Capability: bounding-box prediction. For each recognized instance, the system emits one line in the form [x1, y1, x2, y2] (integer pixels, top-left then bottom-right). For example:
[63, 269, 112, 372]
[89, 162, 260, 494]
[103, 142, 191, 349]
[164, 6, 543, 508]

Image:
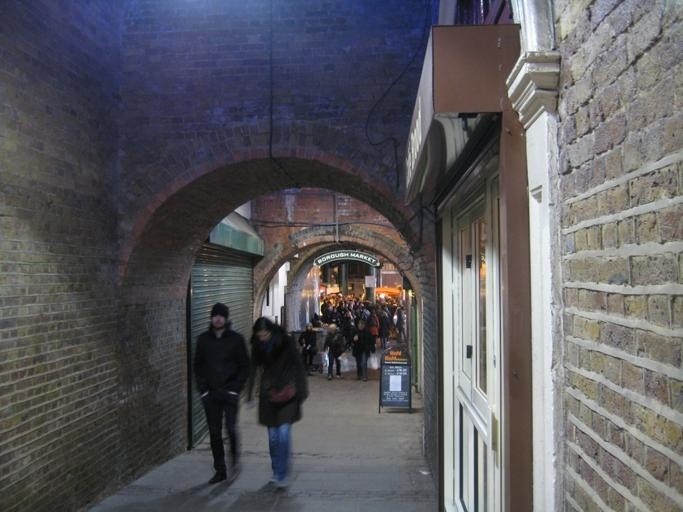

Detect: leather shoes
[210, 471, 227, 483]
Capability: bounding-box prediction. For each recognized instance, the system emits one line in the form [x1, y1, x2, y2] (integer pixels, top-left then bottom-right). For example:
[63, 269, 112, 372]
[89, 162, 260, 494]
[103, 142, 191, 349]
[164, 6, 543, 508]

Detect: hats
[211, 302, 229, 319]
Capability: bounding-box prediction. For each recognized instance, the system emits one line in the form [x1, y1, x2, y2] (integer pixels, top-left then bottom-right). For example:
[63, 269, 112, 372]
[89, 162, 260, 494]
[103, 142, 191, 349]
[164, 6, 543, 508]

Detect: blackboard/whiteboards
[380, 361, 410, 406]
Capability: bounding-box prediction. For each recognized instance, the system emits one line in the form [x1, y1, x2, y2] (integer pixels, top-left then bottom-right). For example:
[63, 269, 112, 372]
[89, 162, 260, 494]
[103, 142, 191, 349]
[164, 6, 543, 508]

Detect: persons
[192, 302, 250, 486]
[296, 321, 318, 367]
[244, 316, 309, 490]
[311, 291, 406, 382]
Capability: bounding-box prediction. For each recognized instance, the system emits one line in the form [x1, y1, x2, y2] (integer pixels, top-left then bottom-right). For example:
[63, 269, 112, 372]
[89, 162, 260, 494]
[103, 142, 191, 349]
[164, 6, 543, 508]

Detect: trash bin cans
[292, 328, 324, 371]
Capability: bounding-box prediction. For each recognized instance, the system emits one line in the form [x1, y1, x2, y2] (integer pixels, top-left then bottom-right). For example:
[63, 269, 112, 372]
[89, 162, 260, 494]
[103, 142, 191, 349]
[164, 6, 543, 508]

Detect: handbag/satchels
[268, 384, 297, 403]
